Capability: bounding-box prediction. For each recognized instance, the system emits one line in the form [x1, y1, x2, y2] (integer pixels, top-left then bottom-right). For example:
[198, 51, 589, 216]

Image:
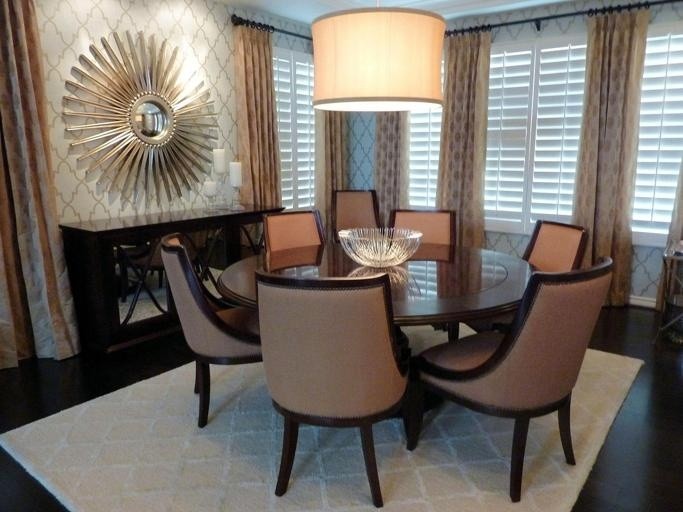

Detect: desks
[62, 201, 282, 368]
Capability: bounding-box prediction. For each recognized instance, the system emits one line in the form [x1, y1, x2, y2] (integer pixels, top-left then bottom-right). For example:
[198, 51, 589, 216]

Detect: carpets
[0, 343, 645, 511]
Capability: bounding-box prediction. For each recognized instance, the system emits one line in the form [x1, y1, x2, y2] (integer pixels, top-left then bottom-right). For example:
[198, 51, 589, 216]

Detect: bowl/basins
[338, 228, 424, 269]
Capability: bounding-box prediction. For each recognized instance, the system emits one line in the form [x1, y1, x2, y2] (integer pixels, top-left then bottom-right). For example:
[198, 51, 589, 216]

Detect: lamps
[308, 0, 451, 118]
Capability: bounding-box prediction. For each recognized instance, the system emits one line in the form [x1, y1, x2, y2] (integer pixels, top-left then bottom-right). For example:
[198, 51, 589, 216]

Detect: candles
[202, 148, 242, 197]
